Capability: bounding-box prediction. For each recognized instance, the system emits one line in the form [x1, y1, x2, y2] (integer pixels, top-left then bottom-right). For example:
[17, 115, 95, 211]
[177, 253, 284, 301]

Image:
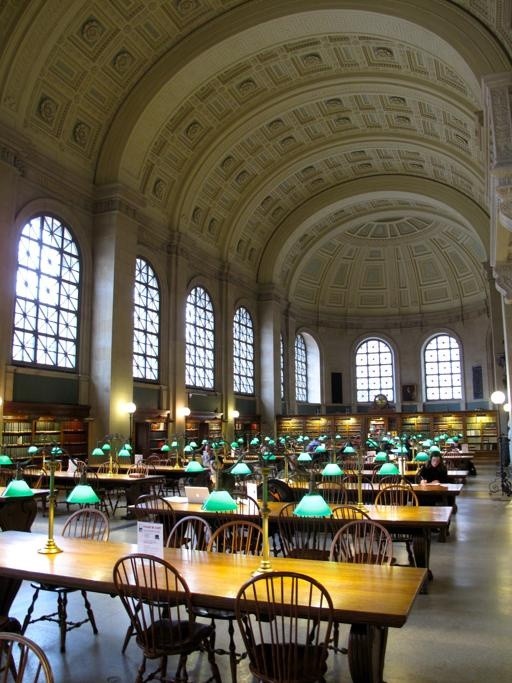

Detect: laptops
[183, 486, 210, 503]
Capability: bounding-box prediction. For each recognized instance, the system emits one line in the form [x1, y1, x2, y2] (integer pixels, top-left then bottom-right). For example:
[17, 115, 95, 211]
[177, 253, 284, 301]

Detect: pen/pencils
[421, 476, 424, 480]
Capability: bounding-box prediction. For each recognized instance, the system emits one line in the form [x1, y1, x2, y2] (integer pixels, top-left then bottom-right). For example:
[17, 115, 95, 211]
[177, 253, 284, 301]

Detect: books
[404, 414, 498, 449]
[3, 421, 60, 485]
[279, 416, 385, 445]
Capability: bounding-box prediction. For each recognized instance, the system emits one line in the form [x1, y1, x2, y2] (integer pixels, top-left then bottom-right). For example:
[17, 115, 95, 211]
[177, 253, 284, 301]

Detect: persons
[190, 454, 235, 552]
[376, 442, 391, 453]
[418, 449, 448, 485]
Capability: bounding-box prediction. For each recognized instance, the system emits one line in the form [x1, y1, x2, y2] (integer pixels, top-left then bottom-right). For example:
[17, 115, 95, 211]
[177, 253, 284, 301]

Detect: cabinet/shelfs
[1, 401, 92, 485]
[136, 407, 260, 458]
[278, 409, 500, 458]
